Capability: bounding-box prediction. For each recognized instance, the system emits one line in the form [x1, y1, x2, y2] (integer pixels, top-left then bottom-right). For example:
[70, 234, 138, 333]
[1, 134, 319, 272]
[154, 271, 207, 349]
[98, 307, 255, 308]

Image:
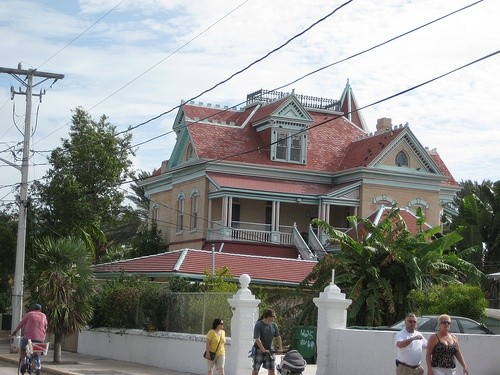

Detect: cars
[390, 314, 497, 335]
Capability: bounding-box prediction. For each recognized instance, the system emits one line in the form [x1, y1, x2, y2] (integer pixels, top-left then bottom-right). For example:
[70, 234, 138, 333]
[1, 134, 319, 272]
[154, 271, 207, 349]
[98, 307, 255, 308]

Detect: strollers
[263, 349, 306, 375]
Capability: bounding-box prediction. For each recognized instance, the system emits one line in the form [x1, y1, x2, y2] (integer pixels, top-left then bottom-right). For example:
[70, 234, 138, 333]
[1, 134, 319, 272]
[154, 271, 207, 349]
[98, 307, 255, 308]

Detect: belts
[396, 359, 420, 369]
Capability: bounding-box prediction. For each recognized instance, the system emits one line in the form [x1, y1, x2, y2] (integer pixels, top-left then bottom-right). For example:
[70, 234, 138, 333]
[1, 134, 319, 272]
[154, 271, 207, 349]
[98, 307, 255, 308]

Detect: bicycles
[10, 334, 42, 375]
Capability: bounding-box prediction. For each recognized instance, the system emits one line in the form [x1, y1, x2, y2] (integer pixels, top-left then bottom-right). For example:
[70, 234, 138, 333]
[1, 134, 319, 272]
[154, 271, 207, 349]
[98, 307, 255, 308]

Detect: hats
[33, 304, 41, 310]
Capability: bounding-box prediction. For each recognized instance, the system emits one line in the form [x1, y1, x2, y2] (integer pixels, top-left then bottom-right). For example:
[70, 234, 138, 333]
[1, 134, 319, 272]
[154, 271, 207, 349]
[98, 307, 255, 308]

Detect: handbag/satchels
[204, 351, 216, 361]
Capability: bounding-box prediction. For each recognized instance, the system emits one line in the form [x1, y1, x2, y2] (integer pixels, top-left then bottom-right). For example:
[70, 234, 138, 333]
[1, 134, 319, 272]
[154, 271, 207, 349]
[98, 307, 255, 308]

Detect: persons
[252, 309, 282, 375]
[206, 318, 226, 375]
[10, 304, 48, 373]
[396, 314, 428, 375]
[426, 315, 469, 375]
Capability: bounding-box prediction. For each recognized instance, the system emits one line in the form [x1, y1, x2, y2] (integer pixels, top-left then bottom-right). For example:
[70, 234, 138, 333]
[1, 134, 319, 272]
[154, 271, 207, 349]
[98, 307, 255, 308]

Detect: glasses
[220, 323, 224, 325]
[409, 320, 416, 323]
[440, 321, 451, 325]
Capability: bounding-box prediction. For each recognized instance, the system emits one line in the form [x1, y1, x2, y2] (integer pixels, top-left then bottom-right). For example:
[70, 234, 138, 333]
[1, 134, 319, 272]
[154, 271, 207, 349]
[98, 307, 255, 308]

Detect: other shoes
[20, 364, 27, 373]
